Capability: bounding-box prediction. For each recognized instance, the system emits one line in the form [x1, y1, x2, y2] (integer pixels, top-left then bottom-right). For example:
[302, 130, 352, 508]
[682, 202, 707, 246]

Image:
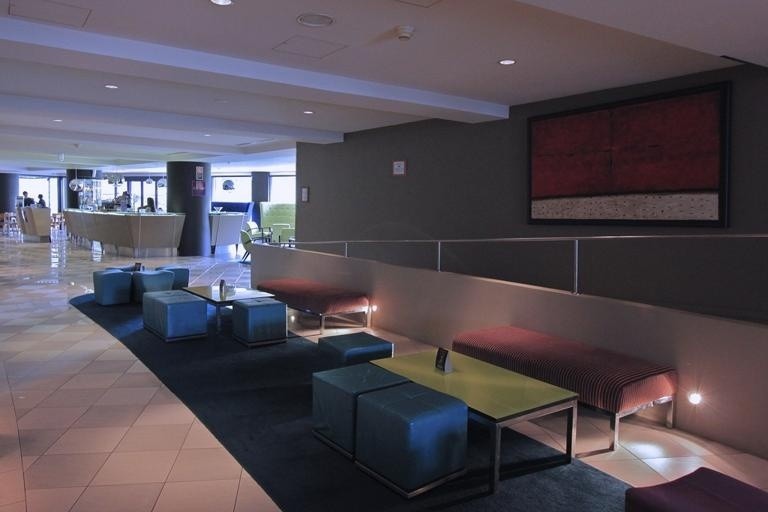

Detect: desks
[15, 207, 28, 235]
[22, 207, 50, 243]
[63, 208, 185, 258]
[209, 212, 243, 253]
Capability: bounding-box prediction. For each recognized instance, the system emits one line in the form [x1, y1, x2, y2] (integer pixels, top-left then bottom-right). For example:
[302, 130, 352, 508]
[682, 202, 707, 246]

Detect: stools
[142, 290, 192, 333]
[155, 265, 189, 291]
[310, 362, 410, 461]
[354, 381, 468, 498]
[231, 297, 287, 348]
[132, 270, 174, 305]
[105, 266, 145, 272]
[318, 332, 394, 368]
[152, 295, 208, 343]
[93, 269, 133, 306]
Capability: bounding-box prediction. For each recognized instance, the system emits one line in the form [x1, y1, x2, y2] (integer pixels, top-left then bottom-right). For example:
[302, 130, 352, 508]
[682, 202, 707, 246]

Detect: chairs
[278, 228, 295, 245]
[246, 221, 271, 243]
[271, 224, 290, 243]
[240, 230, 272, 263]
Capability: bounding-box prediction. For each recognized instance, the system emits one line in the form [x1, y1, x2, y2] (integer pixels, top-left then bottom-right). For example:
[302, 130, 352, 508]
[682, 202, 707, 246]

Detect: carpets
[69, 288, 631, 512]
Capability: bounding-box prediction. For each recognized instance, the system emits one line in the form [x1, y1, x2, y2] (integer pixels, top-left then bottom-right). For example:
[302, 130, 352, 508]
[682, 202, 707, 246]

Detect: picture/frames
[526, 85, 731, 229]
[390, 159, 407, 177]
[300, 185, 310, 203]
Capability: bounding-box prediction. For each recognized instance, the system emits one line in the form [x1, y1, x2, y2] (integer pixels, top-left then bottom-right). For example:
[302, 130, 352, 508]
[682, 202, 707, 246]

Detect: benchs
[451, 325, 676, 450]
[257, 278, 371, 334]
[625, 467, 767, 512]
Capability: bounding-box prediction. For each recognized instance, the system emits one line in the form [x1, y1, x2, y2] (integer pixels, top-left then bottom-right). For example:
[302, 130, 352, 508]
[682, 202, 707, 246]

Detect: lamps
[158, 172, 168, 187]
[223, 180, 236, 191]
[69, 168, 83, 192]
[146, 174, 152, 184]
[116, 175, 125, 185]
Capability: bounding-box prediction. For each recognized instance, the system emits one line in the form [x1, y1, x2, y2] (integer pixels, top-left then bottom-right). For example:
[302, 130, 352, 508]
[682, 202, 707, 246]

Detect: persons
[23, 191, 33, 205]
[145, 198, 158, 213]
[38, 194, 46, 207]
